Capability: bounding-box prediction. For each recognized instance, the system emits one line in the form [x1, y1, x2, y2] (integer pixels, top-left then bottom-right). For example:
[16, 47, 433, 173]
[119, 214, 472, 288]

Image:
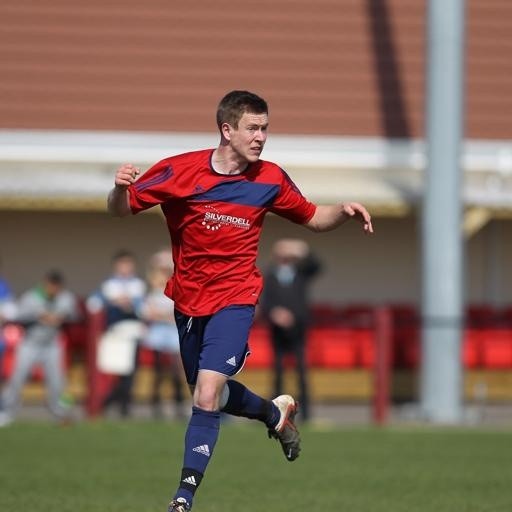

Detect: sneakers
[167, 501, 191, 512]
[268, 394, 302, 460]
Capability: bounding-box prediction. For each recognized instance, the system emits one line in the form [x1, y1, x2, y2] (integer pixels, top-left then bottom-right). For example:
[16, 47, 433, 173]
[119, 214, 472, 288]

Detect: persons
[98, 251, 186, 431]
[261, 239, 323, 426]
[1, 270, 79, 427]
[106, 89, 376, 512]
